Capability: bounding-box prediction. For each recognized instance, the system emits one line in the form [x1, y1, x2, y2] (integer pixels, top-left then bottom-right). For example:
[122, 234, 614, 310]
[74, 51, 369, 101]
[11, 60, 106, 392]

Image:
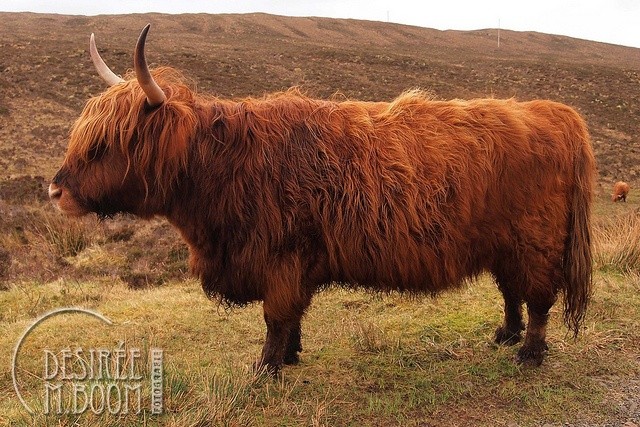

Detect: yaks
[47, 25, 594, 373]
[612, 180, 628, 202]
